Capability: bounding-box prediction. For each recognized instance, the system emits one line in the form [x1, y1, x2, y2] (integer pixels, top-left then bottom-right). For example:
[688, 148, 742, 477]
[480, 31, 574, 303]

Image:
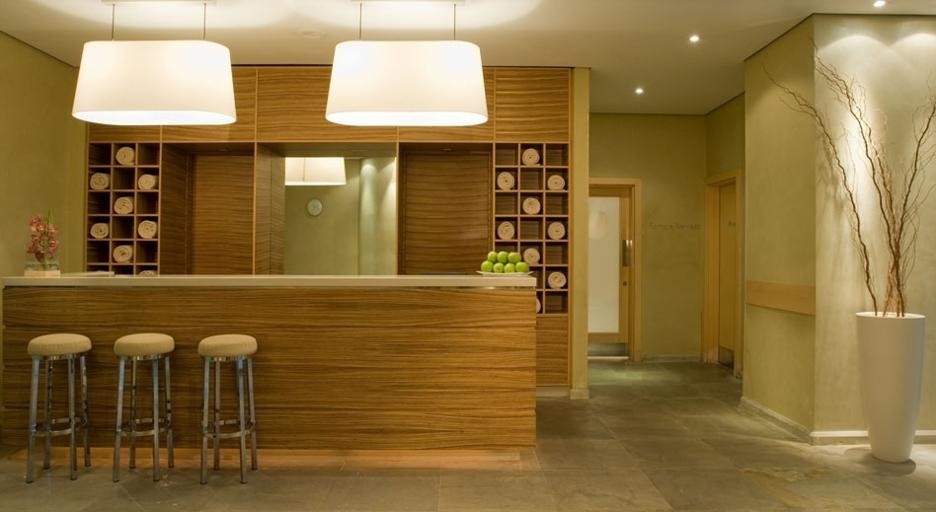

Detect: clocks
[306, 197, 323, 216]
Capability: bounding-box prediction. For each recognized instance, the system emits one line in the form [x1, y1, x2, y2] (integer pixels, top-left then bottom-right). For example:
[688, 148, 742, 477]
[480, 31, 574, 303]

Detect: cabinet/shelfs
[490, 142, 573, 399]
[83, 143, 194, 274]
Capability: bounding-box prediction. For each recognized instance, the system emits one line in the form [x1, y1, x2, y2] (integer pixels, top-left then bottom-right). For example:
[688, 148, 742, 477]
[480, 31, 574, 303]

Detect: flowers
[22, 208, 60, 270]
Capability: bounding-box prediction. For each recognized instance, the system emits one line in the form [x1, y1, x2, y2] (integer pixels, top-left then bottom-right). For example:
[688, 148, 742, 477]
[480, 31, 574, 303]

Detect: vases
[857, 310, 926, 464]
[23, 263, 59, 278]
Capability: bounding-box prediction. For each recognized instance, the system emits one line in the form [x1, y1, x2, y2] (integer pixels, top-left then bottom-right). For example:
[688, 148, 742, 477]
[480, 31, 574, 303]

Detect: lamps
[282, 157, 347, 186]
[69, 2, 237, 125]
[323, 2, 488, 127]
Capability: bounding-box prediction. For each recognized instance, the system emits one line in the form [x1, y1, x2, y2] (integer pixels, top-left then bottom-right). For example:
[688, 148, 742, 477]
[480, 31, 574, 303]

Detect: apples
[480, 250, 529, 273]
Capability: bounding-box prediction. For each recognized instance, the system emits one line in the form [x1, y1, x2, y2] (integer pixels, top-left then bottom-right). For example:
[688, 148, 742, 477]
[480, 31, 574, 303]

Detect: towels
[90, 147, 161, 273]
[496, 147, 568, 312]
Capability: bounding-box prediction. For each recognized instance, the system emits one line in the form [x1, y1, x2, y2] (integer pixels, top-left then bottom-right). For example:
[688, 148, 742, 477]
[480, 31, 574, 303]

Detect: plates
[474, 269, 536, 277]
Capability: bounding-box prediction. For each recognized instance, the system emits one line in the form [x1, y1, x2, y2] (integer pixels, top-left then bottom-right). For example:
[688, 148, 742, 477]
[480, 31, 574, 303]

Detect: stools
[24, 333, 257, 484]
